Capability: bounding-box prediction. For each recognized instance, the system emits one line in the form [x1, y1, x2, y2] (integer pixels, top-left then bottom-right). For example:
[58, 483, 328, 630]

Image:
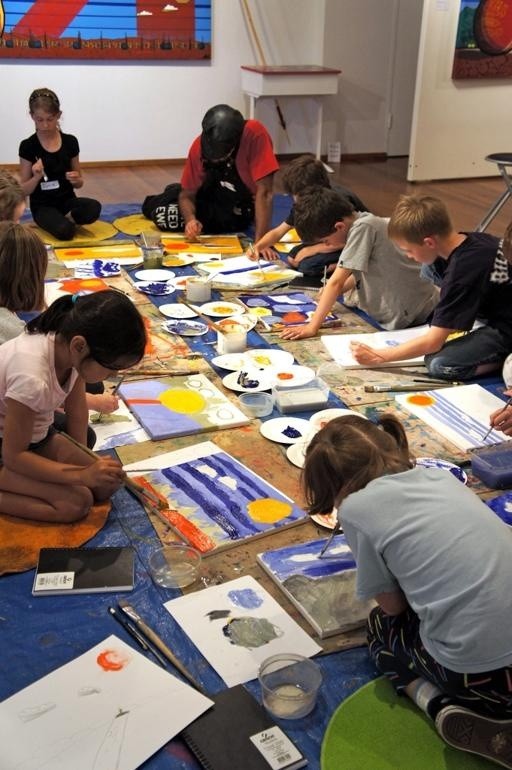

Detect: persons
[0, 219, 104, 452]
[298, 410, 512, 770]
[490, 222, 512, 438]
[142, 105, 282, 261]
[1, 170, 26, 225]
[17, 89, 101, 241]
[0, 289, 146, 524]
[349, 190, 512, 380]
[246, 156, 371, 277]
[278, 186, 441, 342]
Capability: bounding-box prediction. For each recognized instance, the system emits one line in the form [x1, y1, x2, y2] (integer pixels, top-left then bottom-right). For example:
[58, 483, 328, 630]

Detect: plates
[134, 279, 176, 296]
[160, 318, 210, 337]
[135, 268, 175, 281]
[308, 407, 369, 429]
[408, 456, 469, 486]
[200, 300, 245, 318]
[167, 276, 210, 291]
[211, 313, 260, 336]
[285, 442, 313, 469]
[211, 351, 261, 373]
[243, 350, 295, 370]
[261, 364, 317, 390]
[308, 503, 345, 533]
[159, 254, 194, 266]
[259, 415, 315, 444]
[221, 369, 276, 394]
[158, 302, 203, 319]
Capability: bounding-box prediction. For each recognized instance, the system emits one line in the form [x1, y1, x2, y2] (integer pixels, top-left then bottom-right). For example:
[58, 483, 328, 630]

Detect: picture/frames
[0, 0, 212, 62]
[451, 0, 512, 80]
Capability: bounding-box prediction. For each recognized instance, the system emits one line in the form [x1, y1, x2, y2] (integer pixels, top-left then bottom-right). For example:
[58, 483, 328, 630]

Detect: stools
[476, 149, 512, 242]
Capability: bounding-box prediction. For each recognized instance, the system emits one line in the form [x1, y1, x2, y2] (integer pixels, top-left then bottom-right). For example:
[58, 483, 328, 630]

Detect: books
[31, 547, 135, 596]
[184, 685, 309, 768]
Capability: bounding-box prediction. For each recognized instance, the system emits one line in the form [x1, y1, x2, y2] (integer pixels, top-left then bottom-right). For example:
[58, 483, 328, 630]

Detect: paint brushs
[249, 242, 267, 277]
[482, 398, 512, 441]
[211, 285, 267, 293]
[97, 376, 125, 423]
[108, 285, 129, 295]
[204, 272, 220, 284]
[180, 300, 228, 335]
[413, 379, 460, 385]
[195, 235, 206, 244]
[107, 606, 148, 650]
[361, 345, 387, 360]
[118, 599, 205, 694]
[317, 521, 341, 558]
[118, 370, 199, 376]
[34, 155, 49, 182]
[323, 265, 327, 288]
[126, 263, 144, 272]
[260, 330, 283, 334]
[141, 232, 147, 247]
[57, 429, 169, 511]
[365, 385, 453, 393]
[43, 279, 58, 282]
[126, 622, 168, 667]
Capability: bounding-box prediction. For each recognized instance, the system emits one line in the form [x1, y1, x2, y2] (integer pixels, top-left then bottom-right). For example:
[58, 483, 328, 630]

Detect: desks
[238, 65, 342, 173]
